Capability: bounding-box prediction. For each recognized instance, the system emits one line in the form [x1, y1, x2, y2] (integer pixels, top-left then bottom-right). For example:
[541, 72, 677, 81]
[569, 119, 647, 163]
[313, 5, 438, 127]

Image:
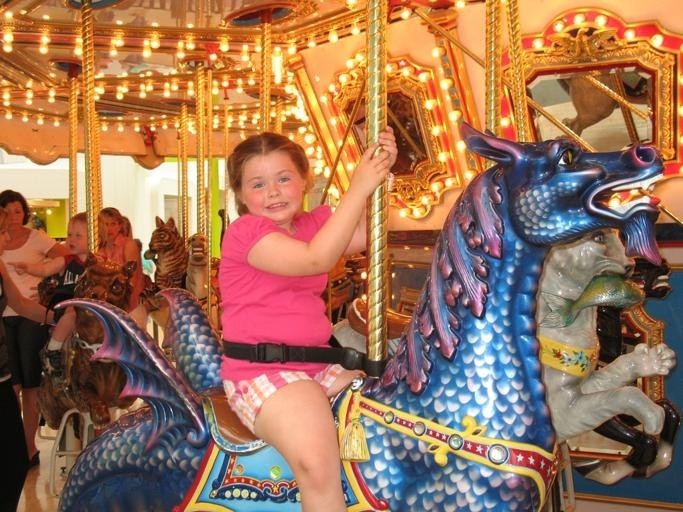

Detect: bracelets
[386, 170, 396, 190]
[44, 308, 50, 324]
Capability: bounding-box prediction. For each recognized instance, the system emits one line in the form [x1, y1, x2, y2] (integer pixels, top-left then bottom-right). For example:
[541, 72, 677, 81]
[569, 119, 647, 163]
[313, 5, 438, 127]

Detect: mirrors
[490, 7, 683, 205]
[326, 48, 462, 219]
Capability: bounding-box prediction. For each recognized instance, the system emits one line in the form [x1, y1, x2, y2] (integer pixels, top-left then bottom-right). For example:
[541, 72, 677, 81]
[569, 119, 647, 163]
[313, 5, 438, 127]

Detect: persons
[7, 212, 108, 373]
[0, 189, 69, 464]
[121, 216, 142, 252]
[216, 125, 400, 512]
[1, 211, 62, 512]
[98, 208, 147, 328]
